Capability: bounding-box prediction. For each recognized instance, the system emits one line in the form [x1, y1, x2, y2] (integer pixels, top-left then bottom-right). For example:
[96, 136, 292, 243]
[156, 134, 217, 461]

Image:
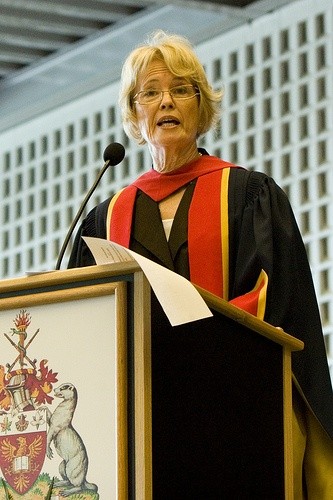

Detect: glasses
[131, 85, 200, 105]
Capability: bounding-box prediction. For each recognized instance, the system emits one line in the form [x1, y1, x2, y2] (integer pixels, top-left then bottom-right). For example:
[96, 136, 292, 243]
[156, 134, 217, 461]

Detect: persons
[66, 28, 333, 500]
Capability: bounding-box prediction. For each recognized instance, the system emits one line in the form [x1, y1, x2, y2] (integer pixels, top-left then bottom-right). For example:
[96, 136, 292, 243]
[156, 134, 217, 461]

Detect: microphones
[56, 142, 126, 271]
